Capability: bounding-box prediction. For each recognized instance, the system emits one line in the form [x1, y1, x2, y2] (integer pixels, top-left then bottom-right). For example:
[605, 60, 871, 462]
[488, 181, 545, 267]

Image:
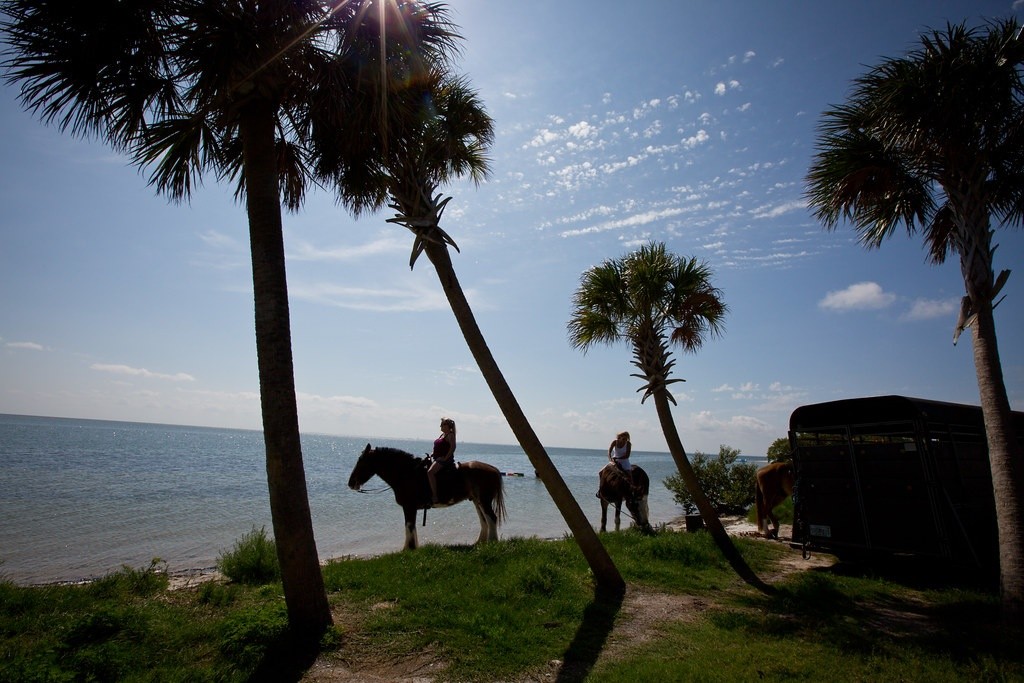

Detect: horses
[755, 459, 793, 538]
[598, 462, 650, 534]
[347, 442, 512, 552]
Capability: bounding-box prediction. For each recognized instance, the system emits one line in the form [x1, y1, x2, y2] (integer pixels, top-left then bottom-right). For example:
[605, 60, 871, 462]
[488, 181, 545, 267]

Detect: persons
[607, 431, 633, 485]
[427, 417, 457, 504]
